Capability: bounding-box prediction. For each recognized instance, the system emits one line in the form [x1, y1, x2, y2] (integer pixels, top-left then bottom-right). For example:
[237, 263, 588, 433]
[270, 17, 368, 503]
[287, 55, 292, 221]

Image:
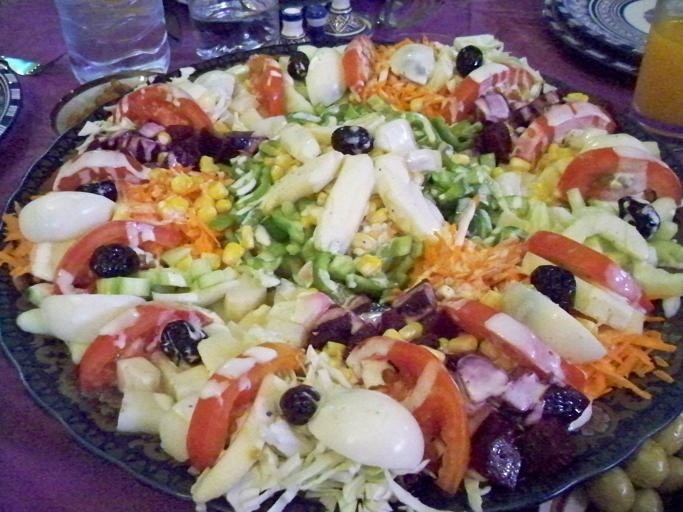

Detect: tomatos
[16, 35, 683, 492]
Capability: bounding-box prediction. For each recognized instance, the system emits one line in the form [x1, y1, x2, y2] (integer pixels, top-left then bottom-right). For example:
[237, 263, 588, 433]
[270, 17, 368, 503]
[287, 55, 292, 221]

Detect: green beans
[586, 414, 682, 509]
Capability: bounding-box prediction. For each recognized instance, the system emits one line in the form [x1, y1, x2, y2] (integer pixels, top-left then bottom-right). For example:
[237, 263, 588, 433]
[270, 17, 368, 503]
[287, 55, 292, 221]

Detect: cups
[626, 0, 683, 138]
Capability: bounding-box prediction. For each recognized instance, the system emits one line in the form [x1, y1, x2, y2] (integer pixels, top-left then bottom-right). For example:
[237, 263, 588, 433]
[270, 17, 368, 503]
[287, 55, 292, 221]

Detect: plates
[543, 0, 654, 73]
[0, 38, 683, 512]
